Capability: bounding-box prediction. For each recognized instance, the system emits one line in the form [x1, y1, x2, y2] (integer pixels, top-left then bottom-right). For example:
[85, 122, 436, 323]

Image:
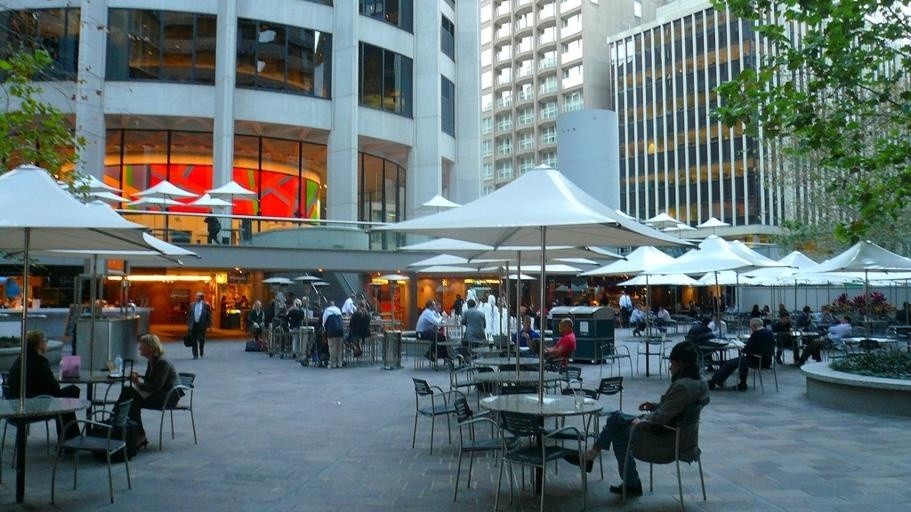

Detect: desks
[271, 317, 402, 367]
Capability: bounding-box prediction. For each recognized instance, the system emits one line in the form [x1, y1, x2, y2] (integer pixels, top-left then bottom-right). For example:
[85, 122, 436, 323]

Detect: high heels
[136, 437, 149, 452]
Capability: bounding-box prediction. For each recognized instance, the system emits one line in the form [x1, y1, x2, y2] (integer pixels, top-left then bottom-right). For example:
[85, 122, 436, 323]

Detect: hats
[661, 340, 699, 366]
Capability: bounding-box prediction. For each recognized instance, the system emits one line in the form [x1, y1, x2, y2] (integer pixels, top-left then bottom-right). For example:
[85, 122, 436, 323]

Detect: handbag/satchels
[184, 329, 193, 347]
[246, 338, 260, 352]
[89, 414, 140, 465]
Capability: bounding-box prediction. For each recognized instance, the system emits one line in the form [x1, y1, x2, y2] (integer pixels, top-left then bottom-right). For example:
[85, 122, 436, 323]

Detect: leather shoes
[707, 379, 725, 389]
[559, 446, 595, 476]
[732, 383, 748, 391]
[608, 481, 646, 498]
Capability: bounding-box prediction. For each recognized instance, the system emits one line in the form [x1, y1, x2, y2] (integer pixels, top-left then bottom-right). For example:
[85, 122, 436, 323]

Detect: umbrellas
[53, 174, 257, 246]
[0, 162, 166, 413]
[18, 200, 200, 375]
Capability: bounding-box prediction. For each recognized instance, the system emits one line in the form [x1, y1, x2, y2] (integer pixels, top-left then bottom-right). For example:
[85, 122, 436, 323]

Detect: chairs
[412, 310, 784, 393]
[0, 372, 197, 505]
[413, 378, 472, 456]
[768, 311, 910, 378]
[618, 399, 707, 510]
[454, 376, 624, 511]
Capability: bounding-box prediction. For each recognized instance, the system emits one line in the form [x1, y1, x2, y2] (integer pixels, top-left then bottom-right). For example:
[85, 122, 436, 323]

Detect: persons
[221, 292, 372, 370]
[107, 333, 185, 450]
[7, 329, 81, 452]
[187, 293, 212, 361]
[560, 341, 709, 496]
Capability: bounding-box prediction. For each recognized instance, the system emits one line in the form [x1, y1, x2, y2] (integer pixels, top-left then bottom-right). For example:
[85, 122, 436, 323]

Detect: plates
[520, 346, 530, 352]
[109, 374, 121, 377]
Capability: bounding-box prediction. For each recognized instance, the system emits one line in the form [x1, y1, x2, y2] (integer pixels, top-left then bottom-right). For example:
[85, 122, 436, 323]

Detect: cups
[443, 308, 463, 324]
[32, 299, 41, 310]
[575, 391, 586, 407]
[489, 343, 494, 348]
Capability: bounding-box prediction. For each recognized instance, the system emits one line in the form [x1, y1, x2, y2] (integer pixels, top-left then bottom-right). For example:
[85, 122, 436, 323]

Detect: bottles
[116, 353, 122, 372]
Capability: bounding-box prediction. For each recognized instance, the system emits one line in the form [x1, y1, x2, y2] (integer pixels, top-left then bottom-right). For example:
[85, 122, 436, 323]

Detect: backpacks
[212, 217, 221, 234]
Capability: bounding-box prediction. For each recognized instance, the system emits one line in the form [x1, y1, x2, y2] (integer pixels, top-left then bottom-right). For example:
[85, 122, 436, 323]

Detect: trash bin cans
[383, 330, 402, 369]
[547, 306, 615, 364]
[724, 306, 738, 315]
[300, 326, 315, 355]
